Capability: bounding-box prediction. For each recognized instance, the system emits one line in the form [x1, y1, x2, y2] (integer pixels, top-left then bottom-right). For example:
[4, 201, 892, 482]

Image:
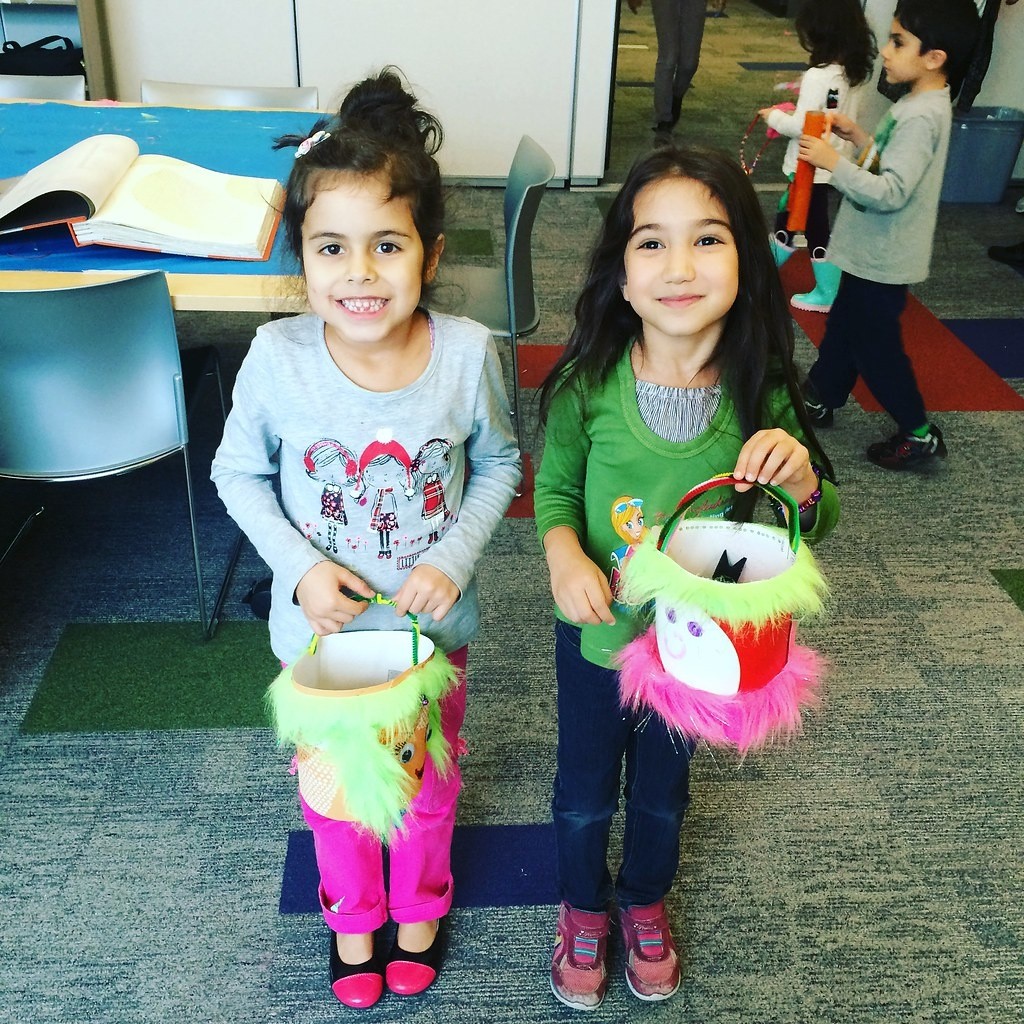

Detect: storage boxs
[938, 105, 1023, 203]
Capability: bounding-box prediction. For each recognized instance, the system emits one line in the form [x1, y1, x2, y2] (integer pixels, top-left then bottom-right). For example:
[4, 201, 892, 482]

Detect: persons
[210, 65, 523, 1006]
[785, 1, 980, 468]
[626, 0, 725, 143]
[532, 148, 838, 1010]
[757, 1, 877, 314]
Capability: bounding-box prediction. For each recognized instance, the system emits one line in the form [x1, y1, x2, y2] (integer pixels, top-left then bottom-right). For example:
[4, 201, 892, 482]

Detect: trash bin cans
[940, 104, 1024, 206]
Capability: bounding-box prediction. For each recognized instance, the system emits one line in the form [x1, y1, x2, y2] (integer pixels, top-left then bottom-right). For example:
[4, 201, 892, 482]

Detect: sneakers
[548, 901, 613, 1009]
[798, 385, 836, 430]
[617, 898, 681, 1001]
[867, 421, 948, 469]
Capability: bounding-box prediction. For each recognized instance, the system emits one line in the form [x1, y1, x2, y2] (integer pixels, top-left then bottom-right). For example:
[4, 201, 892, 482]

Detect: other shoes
[329, 926, 386, 1009]
[650, 94, 685, 146]
[385, 916, 448, 993]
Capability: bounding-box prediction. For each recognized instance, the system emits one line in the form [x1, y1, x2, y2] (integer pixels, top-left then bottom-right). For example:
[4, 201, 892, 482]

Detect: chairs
[0, 271, 251, 641]
[0, 74, 86, 103]
[418, 134, 557, 500]
[139, 78, 320, 112]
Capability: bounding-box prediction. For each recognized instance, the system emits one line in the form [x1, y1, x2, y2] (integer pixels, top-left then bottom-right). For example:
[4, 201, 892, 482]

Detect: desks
[0, 97, 344, 313]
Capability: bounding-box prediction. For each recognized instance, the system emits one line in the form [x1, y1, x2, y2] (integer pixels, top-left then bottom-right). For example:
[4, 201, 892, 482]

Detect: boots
[766, 232, 797, 269]
[789, 255, 844, 313]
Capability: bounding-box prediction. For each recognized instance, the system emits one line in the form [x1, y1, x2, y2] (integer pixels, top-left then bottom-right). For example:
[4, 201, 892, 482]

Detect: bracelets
[769, 462, 823, 517]
